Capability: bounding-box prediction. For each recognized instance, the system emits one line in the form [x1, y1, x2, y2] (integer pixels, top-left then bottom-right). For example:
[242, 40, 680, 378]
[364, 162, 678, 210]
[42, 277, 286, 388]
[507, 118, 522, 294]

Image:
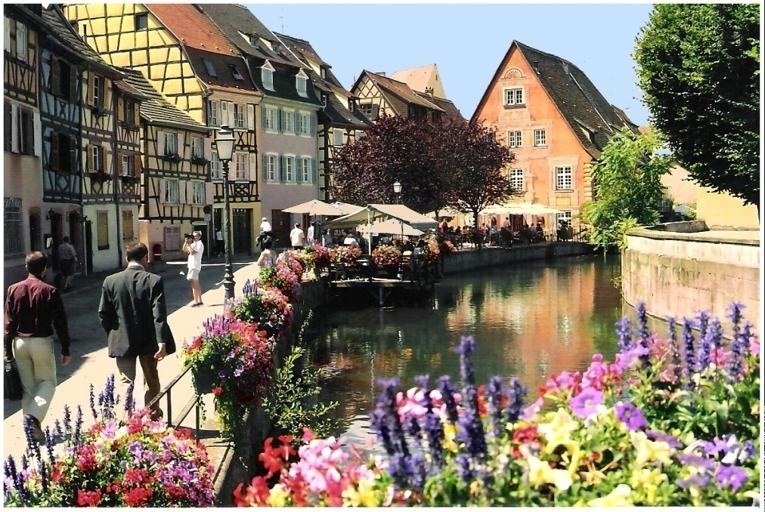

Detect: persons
[97, 241, 177, 423]
[4, 251, 74, 447]
[182, 229, 207, 308]
[214, 229, 227, 259]
[255, 216, 362, 252]
[55, 235, 80, 289]
[438, 217, 569, 247]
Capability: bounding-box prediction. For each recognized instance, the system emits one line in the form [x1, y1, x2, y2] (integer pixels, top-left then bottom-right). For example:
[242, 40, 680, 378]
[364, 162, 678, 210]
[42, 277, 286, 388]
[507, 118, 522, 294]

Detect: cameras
[184, 234, 194, 239]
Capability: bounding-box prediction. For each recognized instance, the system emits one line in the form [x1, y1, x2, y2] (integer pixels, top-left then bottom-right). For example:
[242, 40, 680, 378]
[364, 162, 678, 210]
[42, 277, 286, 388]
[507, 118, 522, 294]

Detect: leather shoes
[23, 414, 45, 446]
[148, 409, 163, 421]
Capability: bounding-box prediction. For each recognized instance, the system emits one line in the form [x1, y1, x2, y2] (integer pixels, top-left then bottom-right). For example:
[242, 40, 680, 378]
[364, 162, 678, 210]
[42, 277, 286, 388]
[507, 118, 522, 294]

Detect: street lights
[213, 124, 239, 310]
[393, 177, 402, 253]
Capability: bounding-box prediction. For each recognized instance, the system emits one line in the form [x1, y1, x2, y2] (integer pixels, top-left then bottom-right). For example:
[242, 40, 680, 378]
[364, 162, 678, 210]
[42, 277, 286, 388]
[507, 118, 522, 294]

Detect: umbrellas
[280, 197, 346, 244]
[309, 200, 366, 217]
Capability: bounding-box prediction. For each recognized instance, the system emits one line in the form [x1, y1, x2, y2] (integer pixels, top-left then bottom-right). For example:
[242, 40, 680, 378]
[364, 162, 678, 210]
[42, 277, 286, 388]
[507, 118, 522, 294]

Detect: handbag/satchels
[4, 355, 22, 400]
